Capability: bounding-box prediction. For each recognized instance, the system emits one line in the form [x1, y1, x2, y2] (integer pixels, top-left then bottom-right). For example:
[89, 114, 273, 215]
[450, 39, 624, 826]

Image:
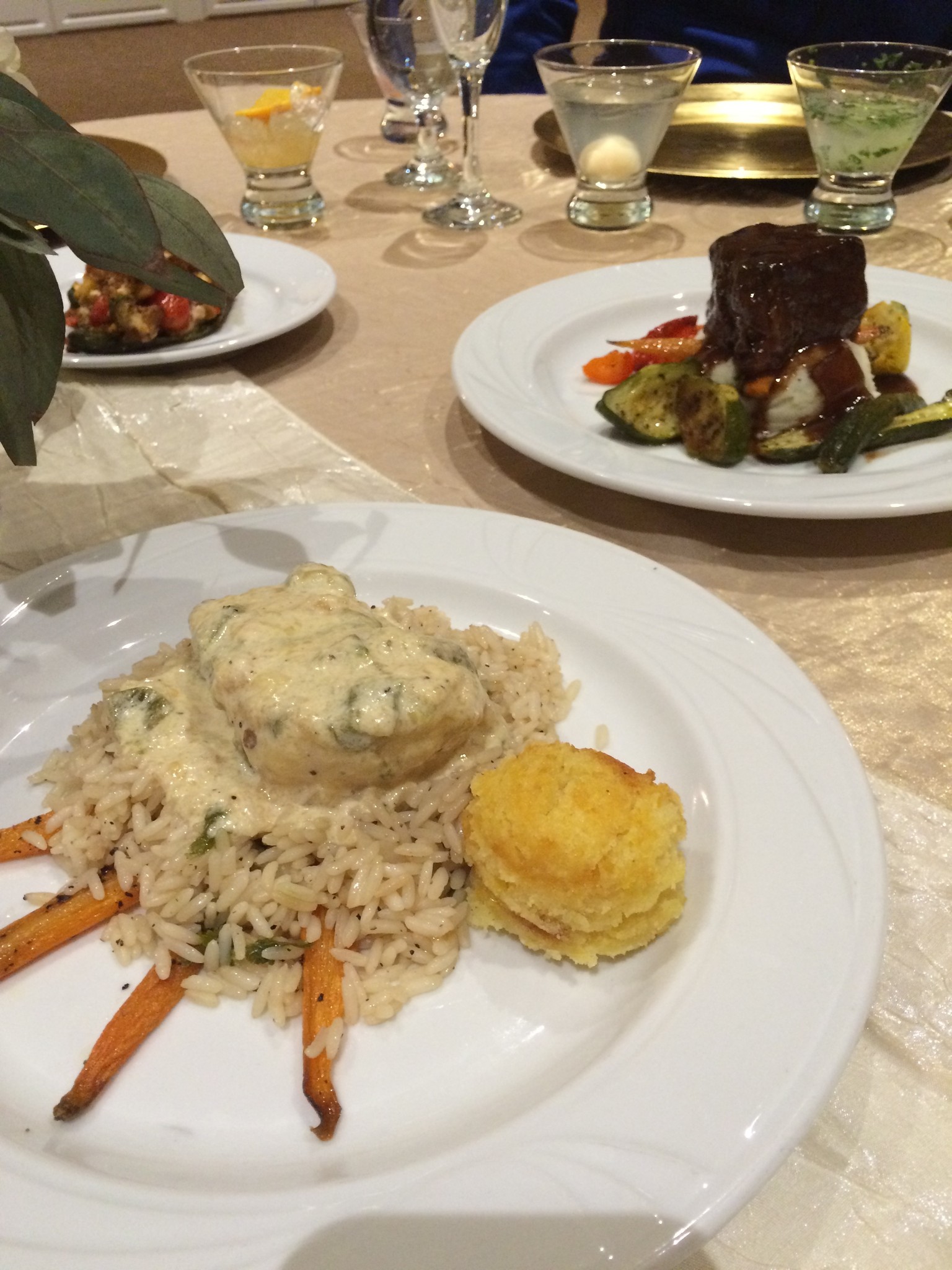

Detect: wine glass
[365, 0, 480, 191]
[417, 0, 525, 231]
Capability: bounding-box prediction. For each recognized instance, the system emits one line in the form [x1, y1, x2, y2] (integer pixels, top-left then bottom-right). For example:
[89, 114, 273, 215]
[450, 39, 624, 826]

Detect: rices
[22, 563, 576, 1025]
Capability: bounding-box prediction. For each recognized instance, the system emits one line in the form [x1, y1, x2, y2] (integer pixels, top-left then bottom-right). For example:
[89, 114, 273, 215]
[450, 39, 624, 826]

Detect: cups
[787, 41, 951, 235]
[345, 2, 446, 138]
[533, 39, 701, 232]
[183, 44, 345, 231]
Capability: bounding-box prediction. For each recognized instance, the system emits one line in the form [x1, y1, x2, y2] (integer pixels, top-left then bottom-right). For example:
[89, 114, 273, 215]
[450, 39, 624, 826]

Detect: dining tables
[4, 92, 950, 1269]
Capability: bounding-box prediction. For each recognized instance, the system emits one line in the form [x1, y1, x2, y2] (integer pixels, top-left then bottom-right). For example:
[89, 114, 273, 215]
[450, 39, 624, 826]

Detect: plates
[0, 497, 886, 1267]
[535, 82, 952, 182]
[45, 233, 338, 370]
[451, 254, 952, 518]
